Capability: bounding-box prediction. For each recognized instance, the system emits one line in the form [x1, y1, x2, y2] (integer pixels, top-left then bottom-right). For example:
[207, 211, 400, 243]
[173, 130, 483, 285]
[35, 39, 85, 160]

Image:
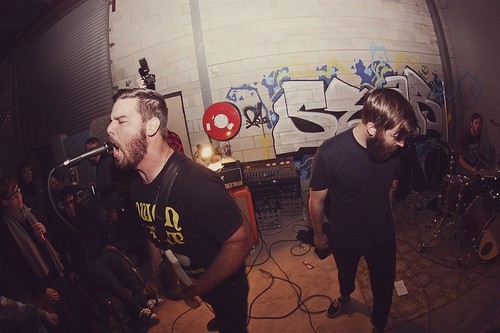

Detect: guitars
[163, 250, 199, 309]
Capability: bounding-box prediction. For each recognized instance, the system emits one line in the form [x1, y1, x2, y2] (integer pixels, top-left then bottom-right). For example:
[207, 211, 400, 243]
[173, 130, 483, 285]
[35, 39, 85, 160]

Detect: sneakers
[371, 326, 385, 333]
[327, 294, 351, 317]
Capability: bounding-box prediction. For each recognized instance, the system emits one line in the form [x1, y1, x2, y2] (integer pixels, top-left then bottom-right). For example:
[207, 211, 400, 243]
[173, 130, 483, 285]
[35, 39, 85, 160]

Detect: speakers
[220, 184, 258, 245]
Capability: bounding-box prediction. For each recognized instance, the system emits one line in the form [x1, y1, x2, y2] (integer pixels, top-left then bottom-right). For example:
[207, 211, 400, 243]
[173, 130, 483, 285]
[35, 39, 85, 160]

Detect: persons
[454, 113, 487, 192]
[0, 131, 185, 333]
[106, 88, 252, 333]
[309, 87, 417, 333]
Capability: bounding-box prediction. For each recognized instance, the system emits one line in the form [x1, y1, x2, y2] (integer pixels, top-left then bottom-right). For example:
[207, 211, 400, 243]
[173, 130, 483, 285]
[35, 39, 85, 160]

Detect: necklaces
[165, 147, 170, 163]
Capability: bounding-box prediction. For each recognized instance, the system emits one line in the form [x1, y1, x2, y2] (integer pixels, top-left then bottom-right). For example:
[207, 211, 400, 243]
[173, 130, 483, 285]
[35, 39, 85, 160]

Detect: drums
[461, 190, 500, 265]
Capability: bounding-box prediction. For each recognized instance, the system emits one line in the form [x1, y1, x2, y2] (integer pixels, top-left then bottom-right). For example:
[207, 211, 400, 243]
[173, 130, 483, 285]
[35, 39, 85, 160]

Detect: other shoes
[137, 307, 157, 322]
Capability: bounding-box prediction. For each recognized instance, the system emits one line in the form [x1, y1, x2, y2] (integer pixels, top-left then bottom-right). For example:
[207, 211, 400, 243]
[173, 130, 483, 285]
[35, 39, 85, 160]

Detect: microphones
[55, 141, 113, 169]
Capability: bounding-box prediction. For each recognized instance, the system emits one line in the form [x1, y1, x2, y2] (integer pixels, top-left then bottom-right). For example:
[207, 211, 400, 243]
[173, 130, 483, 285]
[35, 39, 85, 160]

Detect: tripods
[419, 170, 472, 268]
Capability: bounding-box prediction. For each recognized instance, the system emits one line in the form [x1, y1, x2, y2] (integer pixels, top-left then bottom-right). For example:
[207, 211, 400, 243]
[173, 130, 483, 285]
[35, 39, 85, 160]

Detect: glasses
[0, 187, 21, 201]
